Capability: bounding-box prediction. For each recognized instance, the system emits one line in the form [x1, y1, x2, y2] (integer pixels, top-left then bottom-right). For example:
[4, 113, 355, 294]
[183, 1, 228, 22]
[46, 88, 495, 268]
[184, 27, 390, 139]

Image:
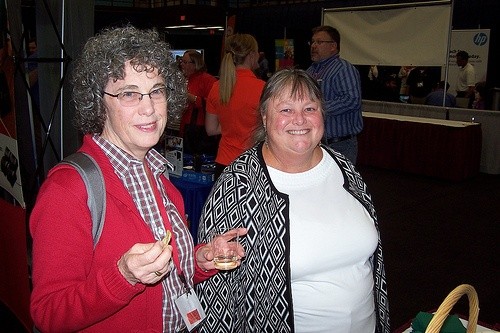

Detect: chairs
[456, 97, 470, 107]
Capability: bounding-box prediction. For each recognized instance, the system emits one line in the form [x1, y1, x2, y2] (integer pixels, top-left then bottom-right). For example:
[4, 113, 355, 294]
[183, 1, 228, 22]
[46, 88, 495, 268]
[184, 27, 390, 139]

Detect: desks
[356, 110, 484, 186]
[390, 307, 500, 333]
[169, 172, 215, 245]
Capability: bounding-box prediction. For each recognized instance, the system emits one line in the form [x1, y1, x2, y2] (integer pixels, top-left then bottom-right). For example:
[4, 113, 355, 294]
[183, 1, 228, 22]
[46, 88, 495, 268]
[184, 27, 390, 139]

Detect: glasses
[182, 60, 194, 65]
[102, 86, 176, 107]
[308, 40, 337, 46]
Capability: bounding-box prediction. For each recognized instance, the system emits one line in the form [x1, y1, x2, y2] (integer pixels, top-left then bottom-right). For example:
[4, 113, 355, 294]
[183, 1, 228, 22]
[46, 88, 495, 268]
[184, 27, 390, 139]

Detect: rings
[155, 271, 163, 276]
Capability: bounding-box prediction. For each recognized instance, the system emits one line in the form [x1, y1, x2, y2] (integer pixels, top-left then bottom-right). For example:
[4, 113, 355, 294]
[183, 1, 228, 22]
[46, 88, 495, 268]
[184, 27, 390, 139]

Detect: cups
[210, 228, 239, 270]
[201, 156, 215, 174]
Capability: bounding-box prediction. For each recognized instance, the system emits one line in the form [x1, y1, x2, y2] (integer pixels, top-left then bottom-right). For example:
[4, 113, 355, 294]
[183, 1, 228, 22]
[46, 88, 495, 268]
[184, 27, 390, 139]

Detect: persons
[30, 27, 247, 333]
[205, 34, 269, 180]
[192, 66, 391, 333]
[26, 40, 42, 115]
[304, 26, 364, 169]
[173, 50, 220, 155]
[398, 48, 478, 107]
